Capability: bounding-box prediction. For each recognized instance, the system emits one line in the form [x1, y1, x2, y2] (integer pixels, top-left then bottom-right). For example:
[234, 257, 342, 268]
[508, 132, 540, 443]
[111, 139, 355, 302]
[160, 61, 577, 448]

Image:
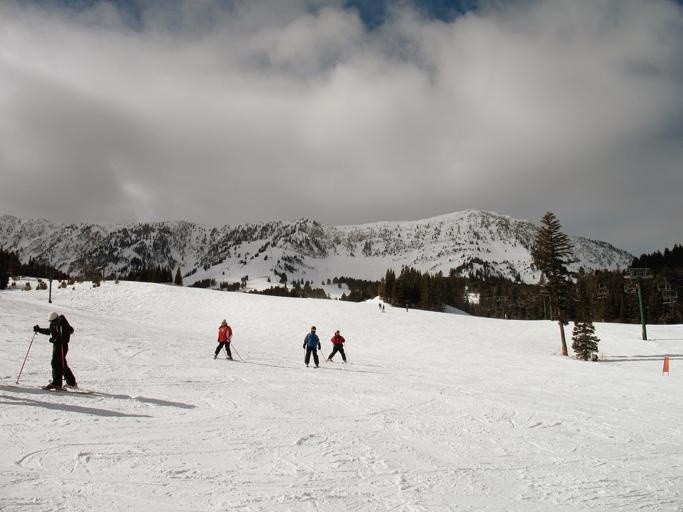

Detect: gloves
[48, 337, 57, 343]
[33, 326, 41, 333]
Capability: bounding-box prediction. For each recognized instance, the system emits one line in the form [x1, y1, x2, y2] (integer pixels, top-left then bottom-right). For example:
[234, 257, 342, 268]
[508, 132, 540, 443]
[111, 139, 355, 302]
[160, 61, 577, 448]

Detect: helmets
[48, 311, 58, 323]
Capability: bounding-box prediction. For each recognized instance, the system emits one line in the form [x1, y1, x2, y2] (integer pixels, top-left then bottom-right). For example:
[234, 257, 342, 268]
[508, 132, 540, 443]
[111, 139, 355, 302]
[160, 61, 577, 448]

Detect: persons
[33, 312, 76, 389]
[328, 330, 346, 362]
[405, 303, 409, 312]
[303, 326, 321, 367]
[378, 303, 385, 312]
[213, 319, 232, 360]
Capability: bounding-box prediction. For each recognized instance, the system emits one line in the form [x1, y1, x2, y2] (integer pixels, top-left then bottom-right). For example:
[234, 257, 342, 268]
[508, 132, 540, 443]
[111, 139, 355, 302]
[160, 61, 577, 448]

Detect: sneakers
[45, 382, 77, 390]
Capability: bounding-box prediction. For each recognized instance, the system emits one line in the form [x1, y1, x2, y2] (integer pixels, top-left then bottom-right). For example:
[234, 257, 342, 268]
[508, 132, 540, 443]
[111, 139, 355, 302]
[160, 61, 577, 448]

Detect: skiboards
[40, 380, 96, 394]
[305, 365, 321, 369]
